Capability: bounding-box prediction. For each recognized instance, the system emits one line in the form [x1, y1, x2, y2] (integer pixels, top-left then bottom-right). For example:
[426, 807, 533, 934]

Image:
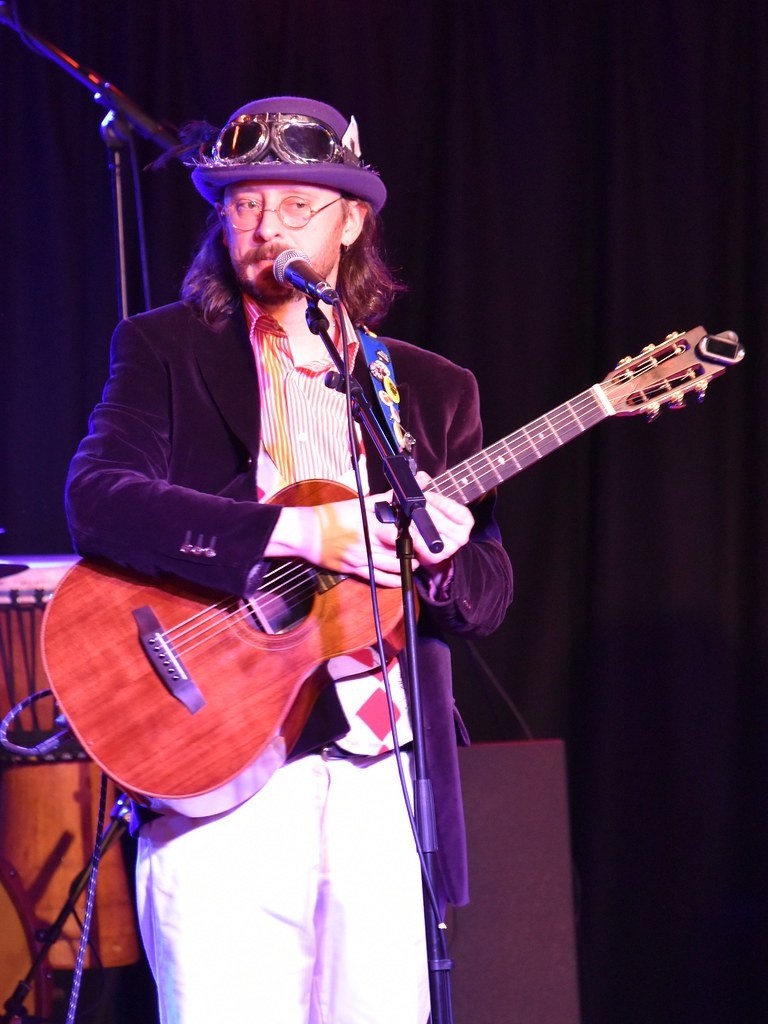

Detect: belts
[309, 740, 414, 761]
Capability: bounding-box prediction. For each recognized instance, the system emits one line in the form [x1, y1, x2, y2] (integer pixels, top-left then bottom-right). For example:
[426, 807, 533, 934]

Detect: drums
[0, 551, 142, 972]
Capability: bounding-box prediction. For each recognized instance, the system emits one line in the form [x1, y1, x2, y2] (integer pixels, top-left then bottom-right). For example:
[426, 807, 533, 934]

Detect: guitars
[38, 320, 750, 823]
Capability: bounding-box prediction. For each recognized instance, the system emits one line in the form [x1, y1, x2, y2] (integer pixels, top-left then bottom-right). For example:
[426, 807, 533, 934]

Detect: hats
[191, 97, 386, 215]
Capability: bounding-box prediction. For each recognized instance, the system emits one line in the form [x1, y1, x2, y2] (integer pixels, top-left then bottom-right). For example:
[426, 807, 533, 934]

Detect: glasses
[221, 196, 343, 231]
[213, 114, 361, 167]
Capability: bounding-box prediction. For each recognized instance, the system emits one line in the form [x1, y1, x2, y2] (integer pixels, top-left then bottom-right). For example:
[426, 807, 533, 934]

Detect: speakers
[0, 744, 149, 987]
[441, 739, 583, 1024]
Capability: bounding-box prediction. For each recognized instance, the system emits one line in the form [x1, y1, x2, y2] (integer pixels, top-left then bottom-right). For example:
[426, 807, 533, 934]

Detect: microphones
[273, 249, 342, 307]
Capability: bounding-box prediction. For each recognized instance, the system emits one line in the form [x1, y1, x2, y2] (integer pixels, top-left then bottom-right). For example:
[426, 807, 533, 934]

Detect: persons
[63, 96, 513, 1024]
